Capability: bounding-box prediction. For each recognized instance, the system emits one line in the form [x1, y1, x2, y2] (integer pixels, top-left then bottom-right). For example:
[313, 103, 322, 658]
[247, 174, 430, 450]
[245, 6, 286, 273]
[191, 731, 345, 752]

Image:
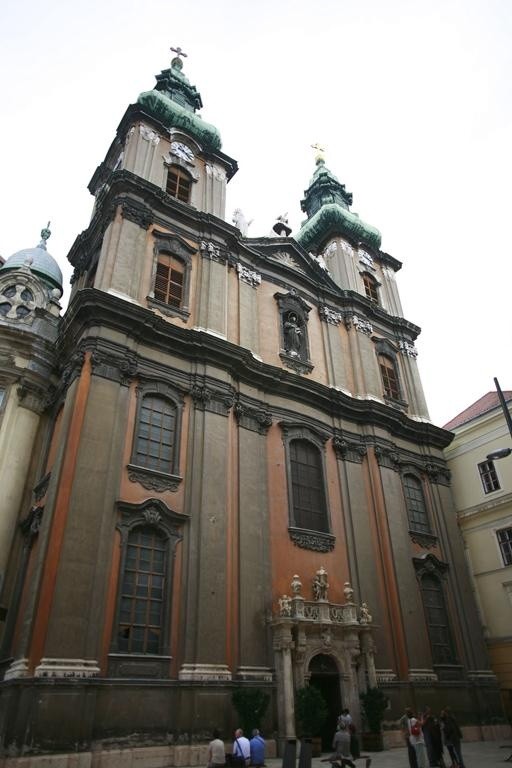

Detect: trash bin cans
[299, 738, 312, 768]
[282, 738, 296, 768]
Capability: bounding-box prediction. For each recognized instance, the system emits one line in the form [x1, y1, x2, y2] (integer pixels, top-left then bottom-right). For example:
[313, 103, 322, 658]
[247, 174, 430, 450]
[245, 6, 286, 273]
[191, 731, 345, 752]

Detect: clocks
[169, 141, 195, 162]
[358, 248, 374, 267]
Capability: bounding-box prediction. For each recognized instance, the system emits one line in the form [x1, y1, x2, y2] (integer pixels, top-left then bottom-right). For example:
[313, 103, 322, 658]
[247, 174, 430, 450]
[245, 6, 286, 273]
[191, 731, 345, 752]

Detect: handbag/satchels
[231, 756, 245, 767]
[412, 725, 419, 736]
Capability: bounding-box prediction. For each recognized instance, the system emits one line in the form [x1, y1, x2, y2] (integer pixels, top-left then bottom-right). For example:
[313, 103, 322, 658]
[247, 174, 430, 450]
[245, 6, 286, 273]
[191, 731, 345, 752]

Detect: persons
[233, 728, 252, 768]
[399, 704, 465, 767]
[330, 708, 360, 765]
[249, 728, 265, 768]
[206, 729, 226, 768]
